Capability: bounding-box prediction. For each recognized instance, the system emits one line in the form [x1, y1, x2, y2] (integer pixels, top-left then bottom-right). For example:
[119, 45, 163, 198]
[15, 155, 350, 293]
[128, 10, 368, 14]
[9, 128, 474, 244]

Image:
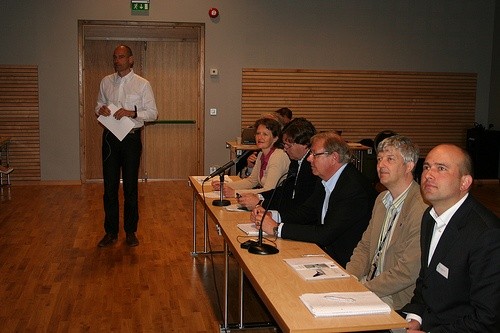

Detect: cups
[237, 137, 241, 145]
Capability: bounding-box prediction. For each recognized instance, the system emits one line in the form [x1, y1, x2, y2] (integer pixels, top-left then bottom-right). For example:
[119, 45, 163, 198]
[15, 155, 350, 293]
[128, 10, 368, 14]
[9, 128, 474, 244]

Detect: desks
[188, 175, 410, 333]
[225, 141, 372, 173]
[0, 137, 10, 185]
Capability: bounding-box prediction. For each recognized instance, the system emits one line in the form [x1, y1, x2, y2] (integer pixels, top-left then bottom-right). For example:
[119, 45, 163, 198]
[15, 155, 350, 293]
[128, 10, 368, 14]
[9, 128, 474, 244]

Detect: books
[236, 223, 268, 236]
[283, 258, 350, 280]
[299, 291, 391, 317]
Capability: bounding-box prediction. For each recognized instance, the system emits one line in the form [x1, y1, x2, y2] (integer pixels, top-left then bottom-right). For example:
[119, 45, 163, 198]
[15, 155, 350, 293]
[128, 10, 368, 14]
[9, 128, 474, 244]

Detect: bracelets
[274, 223, 280, 237]
[131, 105, 137, 119]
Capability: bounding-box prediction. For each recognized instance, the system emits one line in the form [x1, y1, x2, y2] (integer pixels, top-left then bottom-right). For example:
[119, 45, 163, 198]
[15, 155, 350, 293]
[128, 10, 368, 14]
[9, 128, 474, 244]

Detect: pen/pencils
[304, 254, 325, 257]
[236, 193, 243, 199]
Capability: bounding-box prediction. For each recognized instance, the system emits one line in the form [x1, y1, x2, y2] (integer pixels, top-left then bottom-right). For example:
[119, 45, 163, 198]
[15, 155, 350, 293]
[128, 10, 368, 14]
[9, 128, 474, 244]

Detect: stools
[0, 166, 15, 185]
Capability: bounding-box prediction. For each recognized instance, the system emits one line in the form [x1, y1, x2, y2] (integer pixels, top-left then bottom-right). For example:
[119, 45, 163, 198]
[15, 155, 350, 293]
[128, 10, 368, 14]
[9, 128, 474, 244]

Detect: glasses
[278, 142, 296, 151]
[309, 150, 330, 157]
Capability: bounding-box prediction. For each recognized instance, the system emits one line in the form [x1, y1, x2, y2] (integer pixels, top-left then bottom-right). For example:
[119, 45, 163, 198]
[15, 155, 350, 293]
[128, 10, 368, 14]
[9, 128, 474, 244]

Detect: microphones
[248, 170, 292, 255]
[204, 161, 235, 183]
[241, 173, 297, 249]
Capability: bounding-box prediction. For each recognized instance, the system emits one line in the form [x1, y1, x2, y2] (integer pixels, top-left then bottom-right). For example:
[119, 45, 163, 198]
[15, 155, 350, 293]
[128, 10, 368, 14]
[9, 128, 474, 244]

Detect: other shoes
[98, 233, 118, 247]
[125, 232, 139, 247]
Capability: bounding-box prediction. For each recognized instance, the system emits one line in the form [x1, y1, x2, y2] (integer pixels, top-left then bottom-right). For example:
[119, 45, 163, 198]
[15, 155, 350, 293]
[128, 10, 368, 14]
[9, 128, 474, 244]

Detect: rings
[255, 221, 261, 226]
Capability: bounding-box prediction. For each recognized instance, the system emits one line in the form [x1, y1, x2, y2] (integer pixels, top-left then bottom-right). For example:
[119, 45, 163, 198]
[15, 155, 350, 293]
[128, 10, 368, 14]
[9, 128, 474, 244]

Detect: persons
[240, 151, 257, 179]
[250, 131, 378, 269]
[95, 46, 158, 247]
[376, 143, 500, 333]
[235, 117, 326, 225]
[275, 108, 293, 130]
[345, 136, 430, 310]
[211, 119, 292, 198]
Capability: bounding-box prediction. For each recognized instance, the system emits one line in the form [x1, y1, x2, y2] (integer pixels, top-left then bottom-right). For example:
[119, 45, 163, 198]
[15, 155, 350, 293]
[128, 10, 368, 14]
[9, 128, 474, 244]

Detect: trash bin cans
[466, 126, 496, 180]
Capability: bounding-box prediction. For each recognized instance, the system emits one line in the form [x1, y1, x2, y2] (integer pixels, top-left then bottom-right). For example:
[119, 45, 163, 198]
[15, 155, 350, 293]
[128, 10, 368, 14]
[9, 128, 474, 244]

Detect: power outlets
[210, 167, 216, 174]
